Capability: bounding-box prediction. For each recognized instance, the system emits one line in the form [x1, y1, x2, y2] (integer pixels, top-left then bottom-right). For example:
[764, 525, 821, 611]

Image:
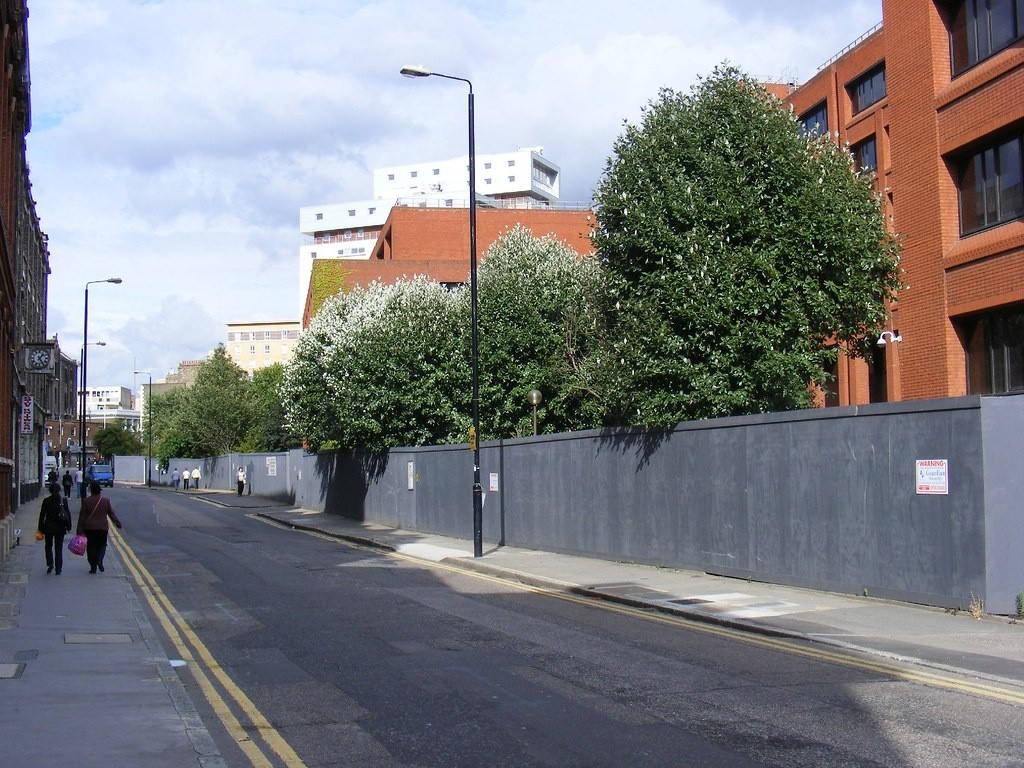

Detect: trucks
[44, 455, 58, 484]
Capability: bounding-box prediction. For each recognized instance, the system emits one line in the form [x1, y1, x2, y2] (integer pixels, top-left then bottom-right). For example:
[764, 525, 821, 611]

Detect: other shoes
[56, 571, 62, 575]
[97, 560, 104, 572]
[47, 565, 54, 573]
[89, 569, 96, 573]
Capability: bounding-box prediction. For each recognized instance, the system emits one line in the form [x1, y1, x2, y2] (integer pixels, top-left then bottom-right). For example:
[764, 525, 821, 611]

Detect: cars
[88, 464, 114, 487]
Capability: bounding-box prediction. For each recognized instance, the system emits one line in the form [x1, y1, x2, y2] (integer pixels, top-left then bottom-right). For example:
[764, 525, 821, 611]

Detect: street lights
[76, 342, 107, 496]
[79, 277, 122, 514]
[399, 64, 484, 559]
[134, 371, 152, 487]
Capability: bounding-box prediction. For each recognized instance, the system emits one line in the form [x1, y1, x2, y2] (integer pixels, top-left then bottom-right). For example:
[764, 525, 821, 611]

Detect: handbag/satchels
[242, 477, 247, 486]
[68, 533, 88, 557]
[58, 495, 71, 530]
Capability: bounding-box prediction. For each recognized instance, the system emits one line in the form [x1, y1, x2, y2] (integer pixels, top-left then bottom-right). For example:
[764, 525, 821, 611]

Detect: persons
[237, 467, 246, 496]
[171, 468, 180, 490]
[75, 467, 83, 497]
[62, 470, 73, 499]
[38, 483, 72, 575]
[76, 482, 122, 573]
[182, 468, 190, 490]
[192, 467, 201, 489]
[49, 468, 57, 484]
